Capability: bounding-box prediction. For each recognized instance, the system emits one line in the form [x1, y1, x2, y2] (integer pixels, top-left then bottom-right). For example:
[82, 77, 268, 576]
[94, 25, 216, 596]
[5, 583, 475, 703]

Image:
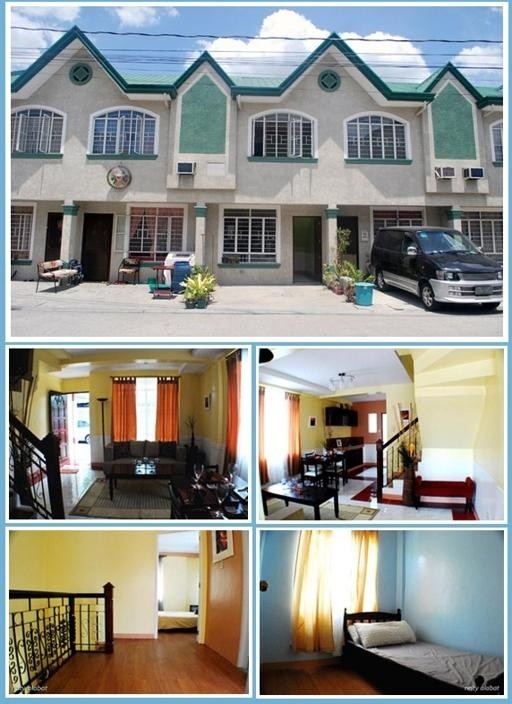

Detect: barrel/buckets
[354, 282, 375, 305]
[171, 261, 191, 294]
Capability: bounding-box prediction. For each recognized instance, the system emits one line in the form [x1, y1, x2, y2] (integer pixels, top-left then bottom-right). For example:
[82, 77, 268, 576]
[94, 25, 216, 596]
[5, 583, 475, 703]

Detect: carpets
[68, 477, 173, 518]
[263, 497, 380, 520]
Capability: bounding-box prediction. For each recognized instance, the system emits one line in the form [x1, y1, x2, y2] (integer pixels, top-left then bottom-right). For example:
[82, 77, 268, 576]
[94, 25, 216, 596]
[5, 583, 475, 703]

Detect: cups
[281, 477, 310, 495]
[311, 448, 337, 459]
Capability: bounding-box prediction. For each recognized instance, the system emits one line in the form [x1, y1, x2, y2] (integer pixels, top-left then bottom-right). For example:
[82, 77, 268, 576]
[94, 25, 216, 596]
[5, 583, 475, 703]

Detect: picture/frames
[212, 530, 234, 563]
[204, 392, 210, 409]
[309, 416, 317, 428]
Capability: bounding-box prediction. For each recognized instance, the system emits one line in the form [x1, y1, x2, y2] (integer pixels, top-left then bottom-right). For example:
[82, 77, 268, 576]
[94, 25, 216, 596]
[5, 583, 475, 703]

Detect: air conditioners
[434, 166, 485, 180]
[176, 160, 196, 174]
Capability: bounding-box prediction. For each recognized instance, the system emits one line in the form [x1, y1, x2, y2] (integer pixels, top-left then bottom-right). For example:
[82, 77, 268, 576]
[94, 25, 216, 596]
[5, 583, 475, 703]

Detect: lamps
[328, 372, 359, 392]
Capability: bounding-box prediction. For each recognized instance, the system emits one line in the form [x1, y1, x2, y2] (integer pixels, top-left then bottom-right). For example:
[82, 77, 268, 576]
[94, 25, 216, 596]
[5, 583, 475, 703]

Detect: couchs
[104, 440, 184, 477]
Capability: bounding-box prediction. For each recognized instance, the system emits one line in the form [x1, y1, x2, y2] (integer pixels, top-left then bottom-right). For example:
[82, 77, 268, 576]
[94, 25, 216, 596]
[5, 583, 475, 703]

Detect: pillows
[347, 620, 416, 648]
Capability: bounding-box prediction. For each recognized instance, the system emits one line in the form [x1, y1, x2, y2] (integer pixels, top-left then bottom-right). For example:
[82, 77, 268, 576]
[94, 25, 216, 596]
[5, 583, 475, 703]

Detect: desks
[261, 482, 339, 520]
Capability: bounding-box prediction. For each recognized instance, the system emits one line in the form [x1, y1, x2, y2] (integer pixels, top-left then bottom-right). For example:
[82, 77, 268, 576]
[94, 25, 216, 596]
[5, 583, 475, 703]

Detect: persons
[216, 530, 227, 552]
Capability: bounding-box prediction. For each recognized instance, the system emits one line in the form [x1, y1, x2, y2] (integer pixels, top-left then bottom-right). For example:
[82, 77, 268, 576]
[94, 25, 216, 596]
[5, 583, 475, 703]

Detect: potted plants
[179, 263, 218, 308]
[322, 227, 375, 305]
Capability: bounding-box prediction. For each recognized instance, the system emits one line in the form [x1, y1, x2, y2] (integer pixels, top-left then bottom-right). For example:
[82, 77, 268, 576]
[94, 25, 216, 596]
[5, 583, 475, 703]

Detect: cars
[74, 419, 90, 443]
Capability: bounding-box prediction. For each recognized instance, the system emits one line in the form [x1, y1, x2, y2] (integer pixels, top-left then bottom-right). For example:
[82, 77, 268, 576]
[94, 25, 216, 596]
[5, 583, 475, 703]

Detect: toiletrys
[294, 483, 307, 495]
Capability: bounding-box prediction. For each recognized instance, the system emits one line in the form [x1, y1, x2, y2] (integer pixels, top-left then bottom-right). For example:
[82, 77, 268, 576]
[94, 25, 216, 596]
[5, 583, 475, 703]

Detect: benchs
[36, 259, 80, 292]
[413, 475, 476, 512]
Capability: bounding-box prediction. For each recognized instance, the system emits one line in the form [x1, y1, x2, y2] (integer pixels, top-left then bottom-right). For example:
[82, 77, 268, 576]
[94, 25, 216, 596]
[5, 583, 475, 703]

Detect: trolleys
[150, 264, 176, 300]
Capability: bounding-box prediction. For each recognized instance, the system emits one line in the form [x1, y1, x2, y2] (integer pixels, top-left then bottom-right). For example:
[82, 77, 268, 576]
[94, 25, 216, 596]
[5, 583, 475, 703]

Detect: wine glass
[193, 462, 248, 519]
[132, 457, 160, 472]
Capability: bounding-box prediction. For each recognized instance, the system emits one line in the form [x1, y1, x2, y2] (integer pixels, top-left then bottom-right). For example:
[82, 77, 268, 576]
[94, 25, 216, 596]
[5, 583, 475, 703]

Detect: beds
[344, 607, 504, 694]
[158, 611, 197, 630]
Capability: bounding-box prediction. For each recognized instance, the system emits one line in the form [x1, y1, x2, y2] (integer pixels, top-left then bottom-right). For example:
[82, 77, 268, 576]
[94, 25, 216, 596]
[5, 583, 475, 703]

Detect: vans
[367, 221, 502, 312]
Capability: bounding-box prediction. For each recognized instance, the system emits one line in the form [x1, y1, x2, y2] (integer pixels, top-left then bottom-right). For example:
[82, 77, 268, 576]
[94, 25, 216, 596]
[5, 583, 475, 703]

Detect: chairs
[118, 257, 140, 284]
[300, 449, 348, 489]
[168, 464, 247, 518]
